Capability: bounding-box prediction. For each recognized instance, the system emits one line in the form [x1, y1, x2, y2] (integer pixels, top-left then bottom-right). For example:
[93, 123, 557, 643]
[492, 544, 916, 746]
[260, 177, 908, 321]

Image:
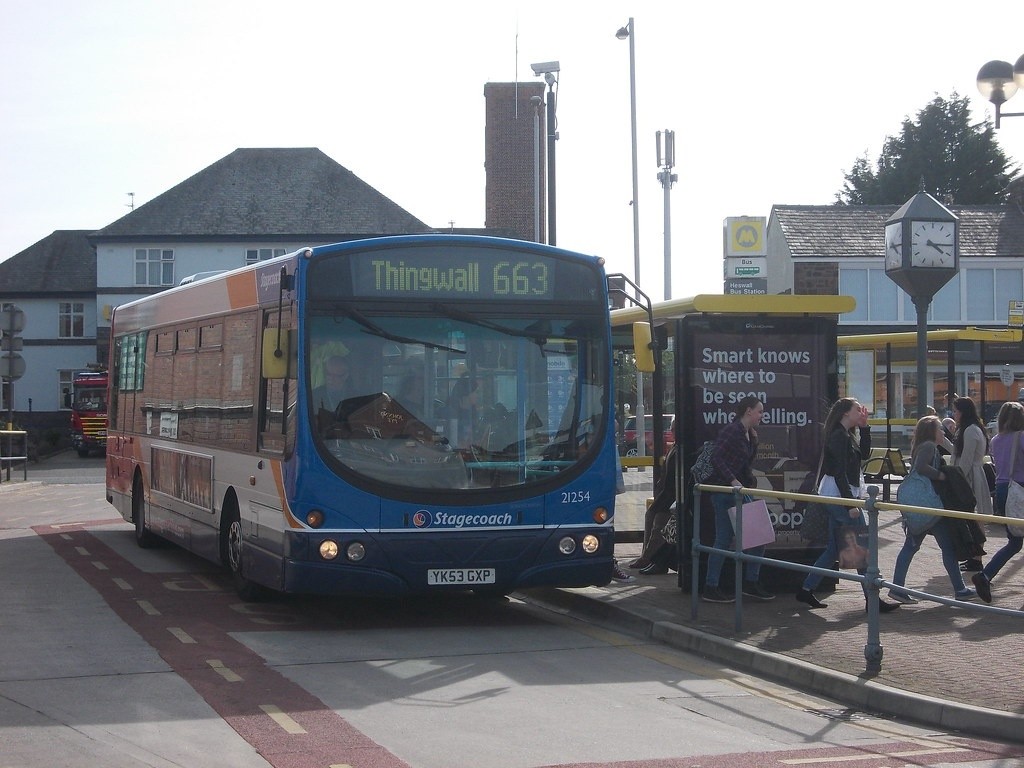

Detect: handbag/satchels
[799, 489, 828, 545]
[729, 494, 775, 551]
[690, 422, 743, 484]
[983, 462, 997, 497]
[834, 517, 870, 569]
[661, 513, 677, 545]
[1005, 480, 1024, 537]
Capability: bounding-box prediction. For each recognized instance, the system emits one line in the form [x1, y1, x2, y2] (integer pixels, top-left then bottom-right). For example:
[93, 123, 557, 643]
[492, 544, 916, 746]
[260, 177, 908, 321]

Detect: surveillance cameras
[531, 61, 560, 76]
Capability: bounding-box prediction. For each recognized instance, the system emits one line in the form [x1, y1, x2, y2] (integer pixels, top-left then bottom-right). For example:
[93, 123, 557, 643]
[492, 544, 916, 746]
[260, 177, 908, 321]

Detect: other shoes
[959, 559, 984, 571]
[629, 557, 648, 568]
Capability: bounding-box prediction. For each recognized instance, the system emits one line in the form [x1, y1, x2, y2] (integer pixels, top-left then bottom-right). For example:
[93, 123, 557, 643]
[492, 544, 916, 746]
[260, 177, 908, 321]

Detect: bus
[106, 234, 659, 604]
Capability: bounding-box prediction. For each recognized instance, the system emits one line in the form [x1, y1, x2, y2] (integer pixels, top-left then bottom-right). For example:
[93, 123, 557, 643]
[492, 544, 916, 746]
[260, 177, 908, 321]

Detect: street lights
[615, 18, 641, 304]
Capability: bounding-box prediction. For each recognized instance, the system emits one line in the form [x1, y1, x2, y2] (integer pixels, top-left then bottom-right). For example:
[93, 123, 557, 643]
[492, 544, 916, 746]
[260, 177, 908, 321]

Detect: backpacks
[898, 441, 944, 545]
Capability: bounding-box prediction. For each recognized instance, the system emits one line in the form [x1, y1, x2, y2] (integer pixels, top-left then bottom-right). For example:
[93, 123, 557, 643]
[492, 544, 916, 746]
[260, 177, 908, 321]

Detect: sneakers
[701, 585, 735, 602]
[742, 581, 777, 599]
[612, 559, 629, 579]
[955, 589, 978, 601]
[888, 588, 918, 604]
[972, 572, 992, 603]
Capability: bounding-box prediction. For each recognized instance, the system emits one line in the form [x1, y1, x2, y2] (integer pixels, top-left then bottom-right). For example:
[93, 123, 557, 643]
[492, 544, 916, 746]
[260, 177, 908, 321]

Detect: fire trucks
[64, 362, 109, 457]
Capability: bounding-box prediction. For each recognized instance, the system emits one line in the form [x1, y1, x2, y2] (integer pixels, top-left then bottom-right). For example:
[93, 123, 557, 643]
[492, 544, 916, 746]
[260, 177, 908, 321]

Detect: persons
[312, 355, 356, 413]
[971, 401, 1024, 613]
[576, 397, 637, 583]
[395, 368, 424, 421]
[939, 396, 993, 572]
[629, 415, 676, 574]
[797, 397, 900, 613]
[887, 416, 978, 604]
[438, 378, 480, 432]
[701, 396, 776, 603]
[924, 405, 956, 456]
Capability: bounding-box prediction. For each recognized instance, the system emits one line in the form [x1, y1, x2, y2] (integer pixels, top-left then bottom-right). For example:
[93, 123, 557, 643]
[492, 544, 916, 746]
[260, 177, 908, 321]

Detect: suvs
[619, 414, 676, 456]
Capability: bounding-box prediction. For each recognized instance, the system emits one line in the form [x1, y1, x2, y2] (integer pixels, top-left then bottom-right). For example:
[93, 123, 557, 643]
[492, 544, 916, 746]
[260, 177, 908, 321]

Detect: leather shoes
[796, 588, 828, 608]
[866, 597, 902, 613]
[639, 562, 668, 575]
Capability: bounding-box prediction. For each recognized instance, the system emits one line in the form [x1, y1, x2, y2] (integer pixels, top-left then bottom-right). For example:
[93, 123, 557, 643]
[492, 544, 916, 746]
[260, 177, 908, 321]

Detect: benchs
[860, 448, 912, 503]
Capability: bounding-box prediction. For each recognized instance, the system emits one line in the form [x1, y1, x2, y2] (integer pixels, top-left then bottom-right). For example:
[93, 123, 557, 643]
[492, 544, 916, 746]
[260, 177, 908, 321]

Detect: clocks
[909, 219, 956, 268]
[884, 222, 903, 270]
